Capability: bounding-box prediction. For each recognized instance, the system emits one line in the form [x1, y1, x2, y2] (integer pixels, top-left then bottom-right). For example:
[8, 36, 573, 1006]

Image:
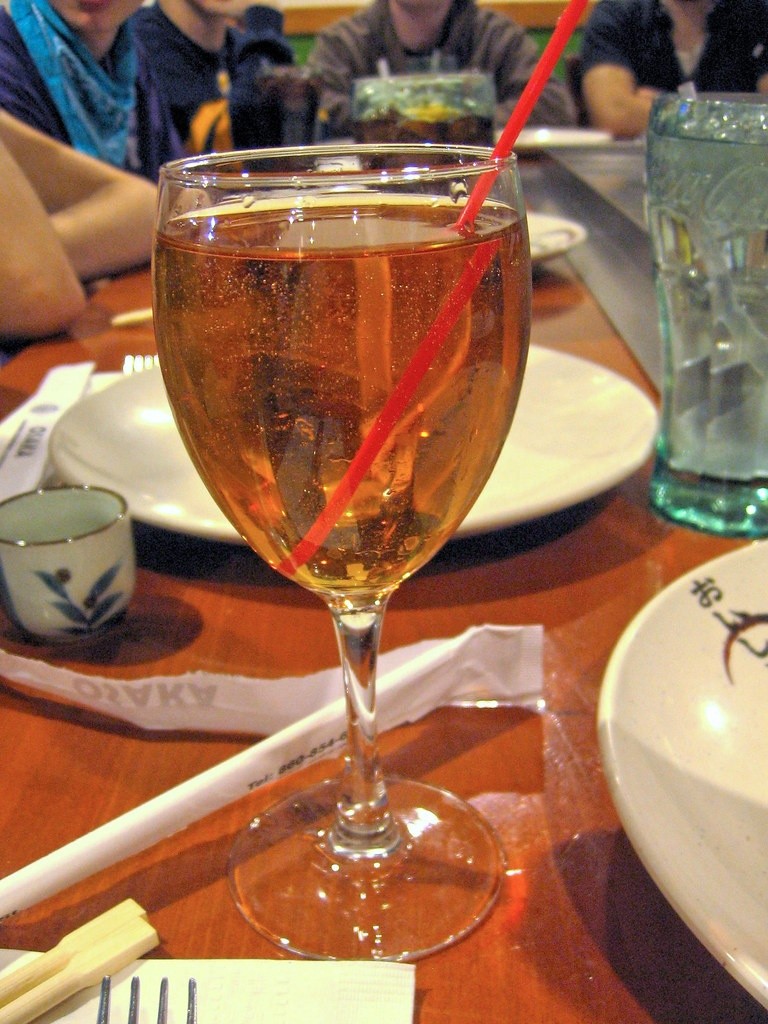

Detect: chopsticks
[0, 899, 159, 1024]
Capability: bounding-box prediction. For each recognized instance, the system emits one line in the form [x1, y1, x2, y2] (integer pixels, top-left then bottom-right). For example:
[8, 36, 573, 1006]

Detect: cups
[0, 483, 137, 643]
[645, 89, 768, 537]
[352, 69, 497, 200]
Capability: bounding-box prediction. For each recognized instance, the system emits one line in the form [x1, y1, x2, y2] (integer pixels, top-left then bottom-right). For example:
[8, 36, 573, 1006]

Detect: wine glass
[150, 142, 535, 962]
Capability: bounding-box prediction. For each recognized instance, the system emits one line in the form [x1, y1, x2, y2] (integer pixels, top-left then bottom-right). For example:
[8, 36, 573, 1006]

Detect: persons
[0, 0, 768, 343]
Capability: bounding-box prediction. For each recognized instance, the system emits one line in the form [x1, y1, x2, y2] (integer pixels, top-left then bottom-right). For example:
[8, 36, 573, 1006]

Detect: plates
[596, 537, 768, 1010]
[47, 347, 662, 547]
[526, 212, 587, 265]
[498, 127, 612, 154]
